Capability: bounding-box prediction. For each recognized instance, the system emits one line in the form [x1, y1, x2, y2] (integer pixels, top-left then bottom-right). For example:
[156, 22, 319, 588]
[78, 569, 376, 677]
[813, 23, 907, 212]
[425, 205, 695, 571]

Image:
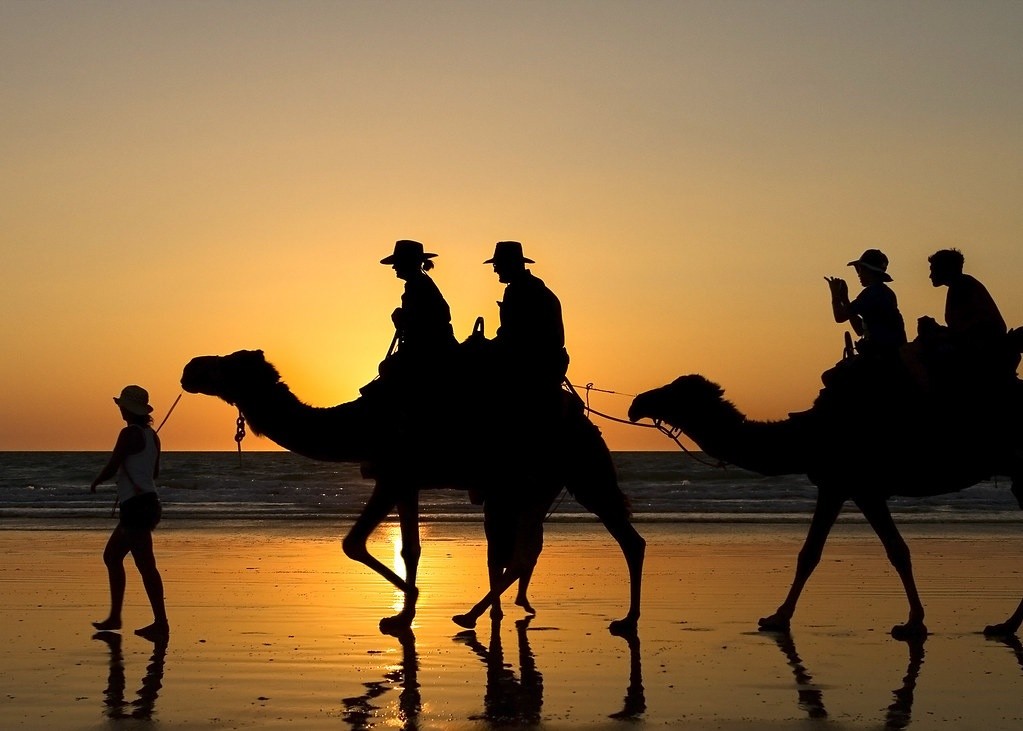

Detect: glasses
[494, 262, 507, 268]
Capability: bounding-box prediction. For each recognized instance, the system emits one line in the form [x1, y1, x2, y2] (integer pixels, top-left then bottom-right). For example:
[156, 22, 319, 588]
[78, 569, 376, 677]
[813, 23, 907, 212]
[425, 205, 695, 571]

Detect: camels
[625, 336, 1023, 642]
[179, 336, 648, 639]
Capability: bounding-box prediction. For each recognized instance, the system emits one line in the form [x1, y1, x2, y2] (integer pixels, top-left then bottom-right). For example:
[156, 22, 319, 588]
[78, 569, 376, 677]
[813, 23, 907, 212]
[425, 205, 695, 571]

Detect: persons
[483, 243, 569, 385]
[822, 250, 908, 355]
[380, 239, 459, 352]
[92, 632, 167, 729]
[919, 249, 1022, 379]
[89, 385, 170, 637]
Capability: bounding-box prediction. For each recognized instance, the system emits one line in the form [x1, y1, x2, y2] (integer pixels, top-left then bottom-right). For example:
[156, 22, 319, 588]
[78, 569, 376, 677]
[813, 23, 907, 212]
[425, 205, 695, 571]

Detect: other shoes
[135, 622, 170, 637]
[93, 619, 123, 630]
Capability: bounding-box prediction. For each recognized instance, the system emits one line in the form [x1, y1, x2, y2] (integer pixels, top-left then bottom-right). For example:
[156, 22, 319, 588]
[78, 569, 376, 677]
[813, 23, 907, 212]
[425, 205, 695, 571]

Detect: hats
[381, 240, 438, 264]
[846, 248, 894, 283]
[113, 385, 153, 412]
[483, 240, 535, 263]
